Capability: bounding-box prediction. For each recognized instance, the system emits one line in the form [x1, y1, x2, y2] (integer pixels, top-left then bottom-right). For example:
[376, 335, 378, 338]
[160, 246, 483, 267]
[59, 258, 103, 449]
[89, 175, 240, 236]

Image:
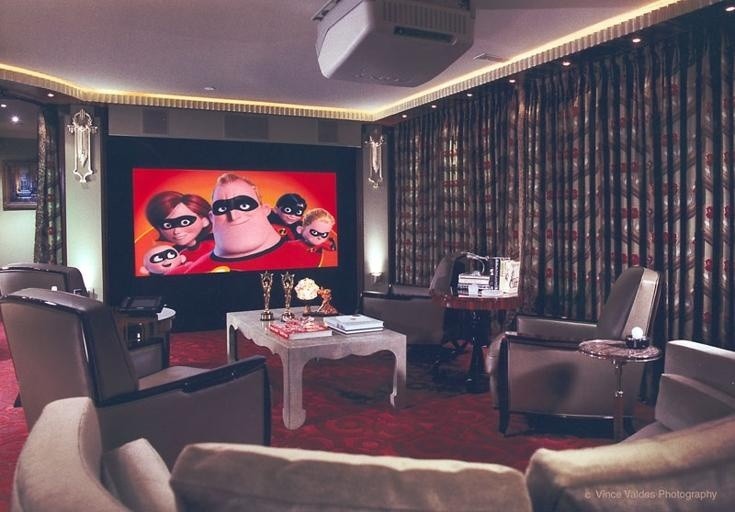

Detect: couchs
[12, 341, 735, 512]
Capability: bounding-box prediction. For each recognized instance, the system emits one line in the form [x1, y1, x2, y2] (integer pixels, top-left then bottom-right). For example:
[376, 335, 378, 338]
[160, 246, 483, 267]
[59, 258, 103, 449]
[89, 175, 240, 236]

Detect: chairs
[1, 262, 89, 297]
[498, 266, 661, 443]
[1, 288, 271, 472]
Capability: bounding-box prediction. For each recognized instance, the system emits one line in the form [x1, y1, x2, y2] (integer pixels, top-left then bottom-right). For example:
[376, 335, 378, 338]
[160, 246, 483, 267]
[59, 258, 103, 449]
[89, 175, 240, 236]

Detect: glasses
[149, 248, 178, 263]
[309, 229, 329, 238]
[160, 215, 197, 230]
[280, 205, 305, 217]
[212, 195, 259, 216]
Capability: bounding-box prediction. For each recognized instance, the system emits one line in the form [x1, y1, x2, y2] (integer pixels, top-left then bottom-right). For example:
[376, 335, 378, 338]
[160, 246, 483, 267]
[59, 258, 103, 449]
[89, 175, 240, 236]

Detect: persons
[139, 173, 337, 275]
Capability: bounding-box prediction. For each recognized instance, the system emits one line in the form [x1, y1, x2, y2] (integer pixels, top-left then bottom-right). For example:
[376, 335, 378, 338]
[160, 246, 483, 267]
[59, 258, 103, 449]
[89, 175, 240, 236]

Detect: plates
[482, 290, 505, 296]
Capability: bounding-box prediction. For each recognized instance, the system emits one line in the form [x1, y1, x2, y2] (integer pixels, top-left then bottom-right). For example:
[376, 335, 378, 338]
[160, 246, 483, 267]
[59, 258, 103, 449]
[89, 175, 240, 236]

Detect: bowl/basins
[626, 335, 650, 348]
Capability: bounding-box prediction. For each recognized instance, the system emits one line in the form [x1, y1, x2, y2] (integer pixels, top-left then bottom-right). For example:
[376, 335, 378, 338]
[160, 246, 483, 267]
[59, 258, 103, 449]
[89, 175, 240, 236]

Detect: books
[456, 255, 522, 299]
[324, 311, 385, 334]
[269, 309, 334, 345]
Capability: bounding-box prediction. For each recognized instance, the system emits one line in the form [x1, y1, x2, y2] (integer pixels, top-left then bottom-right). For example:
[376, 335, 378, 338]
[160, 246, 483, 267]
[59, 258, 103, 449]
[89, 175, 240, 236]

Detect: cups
[467, 285, 478, 295]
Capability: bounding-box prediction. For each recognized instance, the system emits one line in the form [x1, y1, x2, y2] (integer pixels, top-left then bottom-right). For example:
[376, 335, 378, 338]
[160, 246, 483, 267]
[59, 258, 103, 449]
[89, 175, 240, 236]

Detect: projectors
[313, 0, 477, 88]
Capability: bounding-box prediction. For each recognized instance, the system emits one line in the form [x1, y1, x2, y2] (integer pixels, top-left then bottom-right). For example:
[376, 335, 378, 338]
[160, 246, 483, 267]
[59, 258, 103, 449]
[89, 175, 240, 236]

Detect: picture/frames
[3, 157, 39, 210]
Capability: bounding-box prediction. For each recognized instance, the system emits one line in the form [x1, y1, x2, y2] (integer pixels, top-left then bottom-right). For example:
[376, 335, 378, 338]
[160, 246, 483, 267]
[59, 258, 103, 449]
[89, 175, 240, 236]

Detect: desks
[226, 306, 407, 430]
[430, 289, 526, 394]
[112, 306, 177, 368]
[578, 339, 661, 444]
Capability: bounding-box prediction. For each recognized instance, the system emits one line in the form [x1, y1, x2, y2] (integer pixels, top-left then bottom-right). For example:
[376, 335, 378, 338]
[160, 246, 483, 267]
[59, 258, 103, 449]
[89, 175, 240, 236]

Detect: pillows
[169, 442, 532, 512]
[526, 415, 734, 512]
[100, 438, 177, 512]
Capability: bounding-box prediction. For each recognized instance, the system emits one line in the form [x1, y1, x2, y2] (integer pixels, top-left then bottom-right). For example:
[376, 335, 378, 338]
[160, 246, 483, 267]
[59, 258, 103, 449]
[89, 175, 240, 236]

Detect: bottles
[15, 175, 31, 201]
